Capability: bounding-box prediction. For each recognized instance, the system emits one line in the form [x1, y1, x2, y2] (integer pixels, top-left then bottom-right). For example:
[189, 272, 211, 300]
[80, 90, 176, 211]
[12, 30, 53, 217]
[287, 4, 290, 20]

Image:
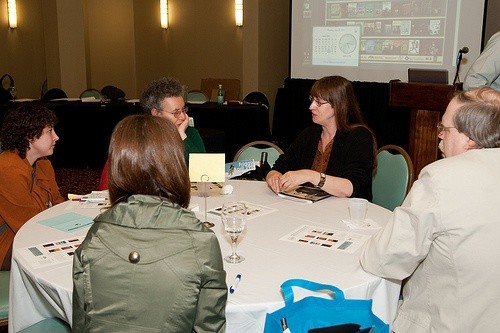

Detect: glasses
[155, 106, 188, 118]
[438, 122, 459, 135]
[309, 95, 330, 107]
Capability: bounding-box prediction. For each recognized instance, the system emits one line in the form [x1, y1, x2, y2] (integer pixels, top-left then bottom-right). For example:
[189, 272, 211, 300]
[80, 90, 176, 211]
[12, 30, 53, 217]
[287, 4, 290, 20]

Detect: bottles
[217, 84, 223, 104]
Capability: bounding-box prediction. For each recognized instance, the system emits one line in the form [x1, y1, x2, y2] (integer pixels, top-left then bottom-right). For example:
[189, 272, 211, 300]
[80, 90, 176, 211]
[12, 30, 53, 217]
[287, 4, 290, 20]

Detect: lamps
[234, 0, 244, 27]
[8, 0, 18, 30]
[159, 0, 168, 29]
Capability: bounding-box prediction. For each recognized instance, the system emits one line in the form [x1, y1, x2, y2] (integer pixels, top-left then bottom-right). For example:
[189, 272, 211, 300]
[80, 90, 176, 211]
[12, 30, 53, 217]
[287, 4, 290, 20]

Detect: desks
[8, 181, 402, 333]
[0, 103, 267, 169]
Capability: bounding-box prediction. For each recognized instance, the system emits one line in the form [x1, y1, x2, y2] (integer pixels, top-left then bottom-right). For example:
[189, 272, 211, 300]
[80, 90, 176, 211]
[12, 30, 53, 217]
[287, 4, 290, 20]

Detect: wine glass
[221, 202, 247, 264]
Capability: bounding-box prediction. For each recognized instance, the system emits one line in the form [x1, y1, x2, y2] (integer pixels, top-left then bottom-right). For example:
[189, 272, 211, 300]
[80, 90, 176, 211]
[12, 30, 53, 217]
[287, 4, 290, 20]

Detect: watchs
[315, 173, 326, 189]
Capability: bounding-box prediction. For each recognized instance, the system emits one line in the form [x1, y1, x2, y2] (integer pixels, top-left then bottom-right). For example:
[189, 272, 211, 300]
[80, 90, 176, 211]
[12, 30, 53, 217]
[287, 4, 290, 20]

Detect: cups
[349, 197, 367, 225]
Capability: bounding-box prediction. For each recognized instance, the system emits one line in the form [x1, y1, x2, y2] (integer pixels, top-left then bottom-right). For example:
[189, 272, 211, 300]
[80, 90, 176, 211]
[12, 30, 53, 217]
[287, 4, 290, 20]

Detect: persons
[72, 114, 228, 333]
[0, 105, 65, 270]
[139, 80, 206, 165]
[463, 31, 500, 91]
[263, 75, 378, 203]
[359, 87, 500, 333]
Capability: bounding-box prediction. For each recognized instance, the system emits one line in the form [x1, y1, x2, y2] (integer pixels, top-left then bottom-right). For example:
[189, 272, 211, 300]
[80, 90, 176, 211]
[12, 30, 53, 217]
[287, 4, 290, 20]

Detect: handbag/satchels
[230, 152, 271, 182]
[264, 279, 390, 333]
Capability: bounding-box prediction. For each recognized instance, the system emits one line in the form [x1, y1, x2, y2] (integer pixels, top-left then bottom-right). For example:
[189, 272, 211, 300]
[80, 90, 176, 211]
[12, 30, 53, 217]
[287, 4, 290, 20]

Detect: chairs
[368, 144, 414, 209]
[79, 89, 101, 98]
[233, 141, 283, 178]
[244, 92, 270, 106]
[187, 90, 208, 102]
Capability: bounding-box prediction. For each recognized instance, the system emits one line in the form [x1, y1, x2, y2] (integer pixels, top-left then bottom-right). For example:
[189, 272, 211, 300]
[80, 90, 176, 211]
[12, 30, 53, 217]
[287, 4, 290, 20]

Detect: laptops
[408, 69, 448, 84]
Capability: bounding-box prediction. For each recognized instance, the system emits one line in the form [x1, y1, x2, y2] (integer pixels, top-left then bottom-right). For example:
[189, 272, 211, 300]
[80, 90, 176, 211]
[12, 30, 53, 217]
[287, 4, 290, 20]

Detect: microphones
[459, 48, 469, 54]
